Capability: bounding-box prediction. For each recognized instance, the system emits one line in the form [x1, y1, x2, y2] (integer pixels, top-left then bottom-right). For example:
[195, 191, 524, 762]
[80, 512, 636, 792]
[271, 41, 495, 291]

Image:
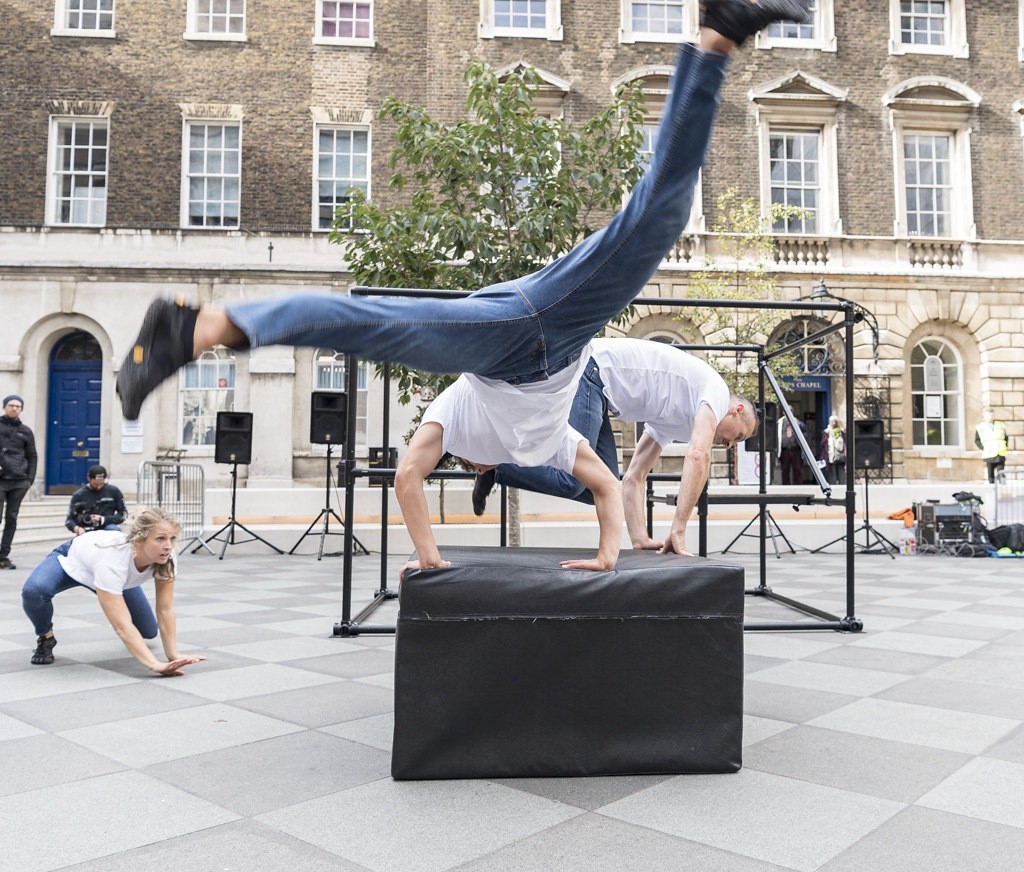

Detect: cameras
[70, 500, 101, 530]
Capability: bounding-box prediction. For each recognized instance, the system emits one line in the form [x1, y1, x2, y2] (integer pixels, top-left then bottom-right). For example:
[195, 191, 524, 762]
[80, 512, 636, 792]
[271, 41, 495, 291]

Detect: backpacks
[825, 429, 846, 464]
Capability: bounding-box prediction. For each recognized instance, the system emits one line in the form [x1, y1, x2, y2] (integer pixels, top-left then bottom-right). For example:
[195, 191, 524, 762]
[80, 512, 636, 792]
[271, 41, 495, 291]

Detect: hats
[3, 395, 24, 412]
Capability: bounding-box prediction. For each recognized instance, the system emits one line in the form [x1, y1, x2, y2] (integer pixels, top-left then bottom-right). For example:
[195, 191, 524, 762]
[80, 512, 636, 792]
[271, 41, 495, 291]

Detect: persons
[64, 465, 129, 532]
[116, 1, 815, 573]
[974, 408, 1009, 488]
[819, 416, 847, 484]
[471, 336, 761, 557]
[21, 507, 199, 677]
[0, 395, 38, 570]
[774, 404, 808, 484]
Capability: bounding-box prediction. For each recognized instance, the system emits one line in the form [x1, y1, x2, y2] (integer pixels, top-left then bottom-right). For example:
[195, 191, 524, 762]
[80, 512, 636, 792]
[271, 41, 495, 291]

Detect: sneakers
[31, 635, 57, 664]
[0, 558, 16, 570]
[116, 297, 188, 420]
[472, 469, 496, 516]
[704, 0, 812, 31]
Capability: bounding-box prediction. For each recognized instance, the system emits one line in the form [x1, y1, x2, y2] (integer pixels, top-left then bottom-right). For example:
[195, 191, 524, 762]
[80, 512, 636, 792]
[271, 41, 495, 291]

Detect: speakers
[744, 402, 778, 452]
[852, 418, 885, 469]
[310, 390, 348, 446]
[214, 411, 253, 464]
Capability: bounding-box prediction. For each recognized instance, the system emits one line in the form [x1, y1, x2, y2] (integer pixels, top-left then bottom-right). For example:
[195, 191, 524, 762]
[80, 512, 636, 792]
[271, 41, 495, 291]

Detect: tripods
[190, 464, 284, 563]
[288, 444, 371, 562]
[720, 509, 798, 560]
[810, 468, 904, 561]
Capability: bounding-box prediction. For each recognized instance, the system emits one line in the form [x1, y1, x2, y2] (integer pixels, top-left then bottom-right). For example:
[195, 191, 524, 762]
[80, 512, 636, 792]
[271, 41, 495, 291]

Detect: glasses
[6, 404, 23, 408]
